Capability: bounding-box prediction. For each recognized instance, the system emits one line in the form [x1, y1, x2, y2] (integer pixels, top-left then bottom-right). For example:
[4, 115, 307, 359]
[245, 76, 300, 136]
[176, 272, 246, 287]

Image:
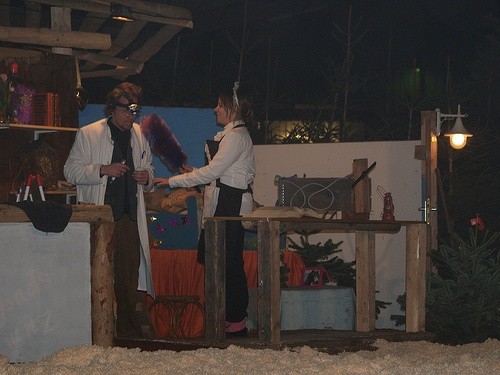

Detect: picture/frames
[299, 267, 323, 288]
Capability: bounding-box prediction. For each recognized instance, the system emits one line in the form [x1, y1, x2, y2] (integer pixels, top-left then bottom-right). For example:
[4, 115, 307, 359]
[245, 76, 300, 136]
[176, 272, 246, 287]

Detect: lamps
[435, 104, 474, 150]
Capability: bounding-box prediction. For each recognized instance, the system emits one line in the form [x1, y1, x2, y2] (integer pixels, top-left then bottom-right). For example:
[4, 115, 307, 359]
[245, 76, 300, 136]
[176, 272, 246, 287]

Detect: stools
[146, 296, 207, 341]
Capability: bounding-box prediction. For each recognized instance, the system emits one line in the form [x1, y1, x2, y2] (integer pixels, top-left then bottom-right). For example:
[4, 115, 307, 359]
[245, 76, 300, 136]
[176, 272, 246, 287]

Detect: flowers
[0, 60, 25, 107]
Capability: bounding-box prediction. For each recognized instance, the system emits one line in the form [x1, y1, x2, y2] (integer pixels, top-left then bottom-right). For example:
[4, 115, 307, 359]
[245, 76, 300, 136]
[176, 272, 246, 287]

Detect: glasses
[112, 101, 141, 115]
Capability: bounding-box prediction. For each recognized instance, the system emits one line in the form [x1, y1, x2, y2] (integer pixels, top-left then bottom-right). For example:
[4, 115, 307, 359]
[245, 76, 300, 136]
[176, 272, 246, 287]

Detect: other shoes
[224, 319, 248, 337]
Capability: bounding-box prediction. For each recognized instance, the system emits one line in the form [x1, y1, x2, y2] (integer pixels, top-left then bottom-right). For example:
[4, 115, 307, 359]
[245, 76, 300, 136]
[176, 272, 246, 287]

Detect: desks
[150, 249, 307, 339]
[203, 216, 431, 350]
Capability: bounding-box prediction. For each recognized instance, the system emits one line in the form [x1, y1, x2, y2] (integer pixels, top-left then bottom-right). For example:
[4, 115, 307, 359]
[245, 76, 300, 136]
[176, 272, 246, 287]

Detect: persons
[63, 82, 157, 338]
[151, 81, 256, 338]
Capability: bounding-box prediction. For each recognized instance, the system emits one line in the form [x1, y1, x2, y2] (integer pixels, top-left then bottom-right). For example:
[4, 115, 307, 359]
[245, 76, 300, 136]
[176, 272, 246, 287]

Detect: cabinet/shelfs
[0, 123, 79, 196]
